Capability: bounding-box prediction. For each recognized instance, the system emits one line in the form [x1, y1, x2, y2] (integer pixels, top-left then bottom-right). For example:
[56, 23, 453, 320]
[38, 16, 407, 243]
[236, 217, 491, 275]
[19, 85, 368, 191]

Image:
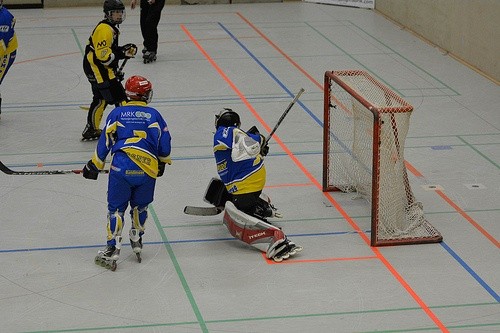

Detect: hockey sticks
[0, 161, 109, 175]
[81, 44, 136, 111]
[183, 88, 305, 216]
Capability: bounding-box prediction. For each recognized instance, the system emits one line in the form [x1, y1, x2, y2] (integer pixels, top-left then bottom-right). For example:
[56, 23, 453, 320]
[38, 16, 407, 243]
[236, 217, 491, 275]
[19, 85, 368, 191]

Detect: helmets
[125, 76, 153, 104]
[215, 108, 240, 130]
[103, 0, 126, 24]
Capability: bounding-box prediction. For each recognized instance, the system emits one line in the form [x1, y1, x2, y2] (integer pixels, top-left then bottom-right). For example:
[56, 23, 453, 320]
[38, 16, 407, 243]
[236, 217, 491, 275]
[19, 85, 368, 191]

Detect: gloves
[261, 135, 269, 157]
[83, 159, 101, 180]
[121, 43, 138, 59]
[157, 160, 166, 177]
[100, 53, 119, 68]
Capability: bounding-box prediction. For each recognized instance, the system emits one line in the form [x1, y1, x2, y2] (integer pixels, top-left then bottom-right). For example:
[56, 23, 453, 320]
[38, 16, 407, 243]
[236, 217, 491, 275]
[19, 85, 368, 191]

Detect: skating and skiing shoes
[273, 239, 302, 263]
[142, 48, 148, 54]
[143, 50, 157, 64]
[82, 122, 103, 141]
[95, 236, 122, 271]
[262, 203, 282, 218]
[129, 228, 142, 263]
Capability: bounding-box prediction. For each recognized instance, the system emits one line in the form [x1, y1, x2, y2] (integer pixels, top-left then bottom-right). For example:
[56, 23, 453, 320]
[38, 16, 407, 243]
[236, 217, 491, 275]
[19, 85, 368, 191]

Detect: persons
[130, 0, 165, 59]
[82, 0, 137, 138]
[204, 107, 295, 258]
[0, 0, 18, 88]
[81, 74, 171, 264]
